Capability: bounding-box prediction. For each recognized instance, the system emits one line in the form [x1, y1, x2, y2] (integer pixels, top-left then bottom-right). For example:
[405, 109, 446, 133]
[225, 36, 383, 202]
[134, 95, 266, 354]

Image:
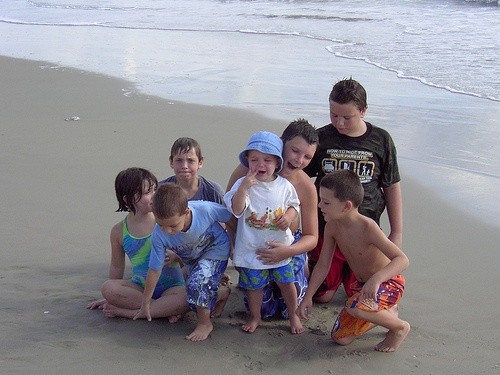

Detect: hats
[239, 131, 283, 175]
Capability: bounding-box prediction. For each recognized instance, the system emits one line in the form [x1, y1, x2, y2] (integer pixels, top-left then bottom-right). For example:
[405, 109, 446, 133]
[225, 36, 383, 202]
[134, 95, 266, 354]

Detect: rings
[283, 221, 286, 225]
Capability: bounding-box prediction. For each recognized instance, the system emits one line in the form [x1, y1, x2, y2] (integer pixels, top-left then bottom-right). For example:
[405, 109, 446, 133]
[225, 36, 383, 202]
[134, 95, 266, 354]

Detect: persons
[86, 167, 192, 323]
[296, 169, 410, 352]
[225, 118, 320, 320]
[223, 131, 305, 334]
[158, 138, 231, 287]
[302, 78, 403, 302]
[134, 183, 238, 342]
